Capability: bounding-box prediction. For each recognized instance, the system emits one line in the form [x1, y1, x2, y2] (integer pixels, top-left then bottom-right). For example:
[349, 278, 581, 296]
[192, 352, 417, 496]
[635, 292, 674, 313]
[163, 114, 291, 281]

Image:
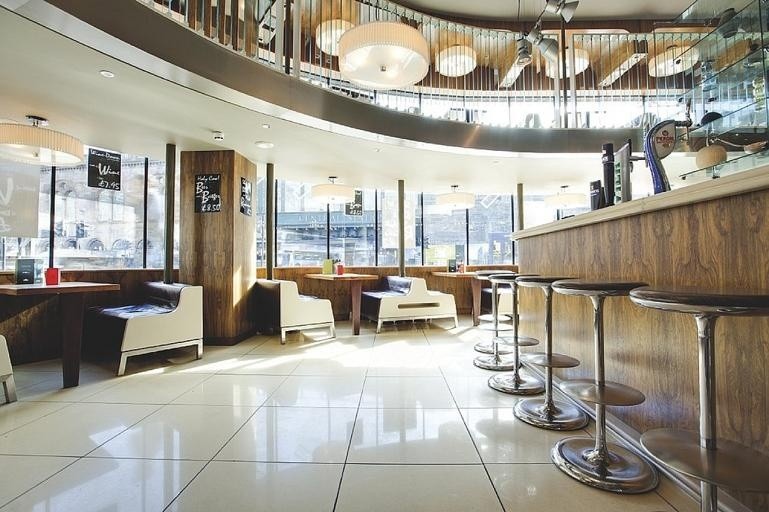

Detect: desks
[431, 272, 482, 325]
[303, 271, 379, 334]
[1, 280, 122, 389]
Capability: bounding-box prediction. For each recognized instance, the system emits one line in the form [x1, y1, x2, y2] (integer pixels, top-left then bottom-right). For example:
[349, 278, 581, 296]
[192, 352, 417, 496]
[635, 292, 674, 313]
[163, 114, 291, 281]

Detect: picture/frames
[194, 174, 221, 213]
[87, 148, 121, 191]
[239, 176, 253, 218]
[344, 191, 364, 217]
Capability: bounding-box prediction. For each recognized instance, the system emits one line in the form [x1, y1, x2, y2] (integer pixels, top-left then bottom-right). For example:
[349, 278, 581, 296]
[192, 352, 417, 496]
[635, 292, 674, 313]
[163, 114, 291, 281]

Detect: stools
[474, 270, 521, 371]
[489, 274, 546, 396]
[552, 279, 661, 493]
[639, 285, 769, 512]
[512, 277, 588, 431]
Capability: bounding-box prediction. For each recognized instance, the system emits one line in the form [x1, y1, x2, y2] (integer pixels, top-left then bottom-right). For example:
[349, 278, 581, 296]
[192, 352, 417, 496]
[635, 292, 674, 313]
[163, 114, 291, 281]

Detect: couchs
[352, 274, 416, 334]
[256, 277, 336, 342]
[0, 333, 19, 405]
[401, 276, 460, 330]
[482, 269, 516, 314]
[83, 280, 205, 377]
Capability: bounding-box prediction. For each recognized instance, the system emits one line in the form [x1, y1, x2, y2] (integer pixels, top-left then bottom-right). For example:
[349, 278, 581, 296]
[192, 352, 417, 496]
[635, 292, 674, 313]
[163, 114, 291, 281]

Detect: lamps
[435, 184, 475, 212]
[435, 44, 476, 78]
[0, 115, 84, 169]
[544, 184, 590, 210]
[336, 21, 432, 93]
[649, 45, 697, 75]
[313, 20, 357, 58]
[310, 176, 355, 205]
[545, 46, 587, 80]
